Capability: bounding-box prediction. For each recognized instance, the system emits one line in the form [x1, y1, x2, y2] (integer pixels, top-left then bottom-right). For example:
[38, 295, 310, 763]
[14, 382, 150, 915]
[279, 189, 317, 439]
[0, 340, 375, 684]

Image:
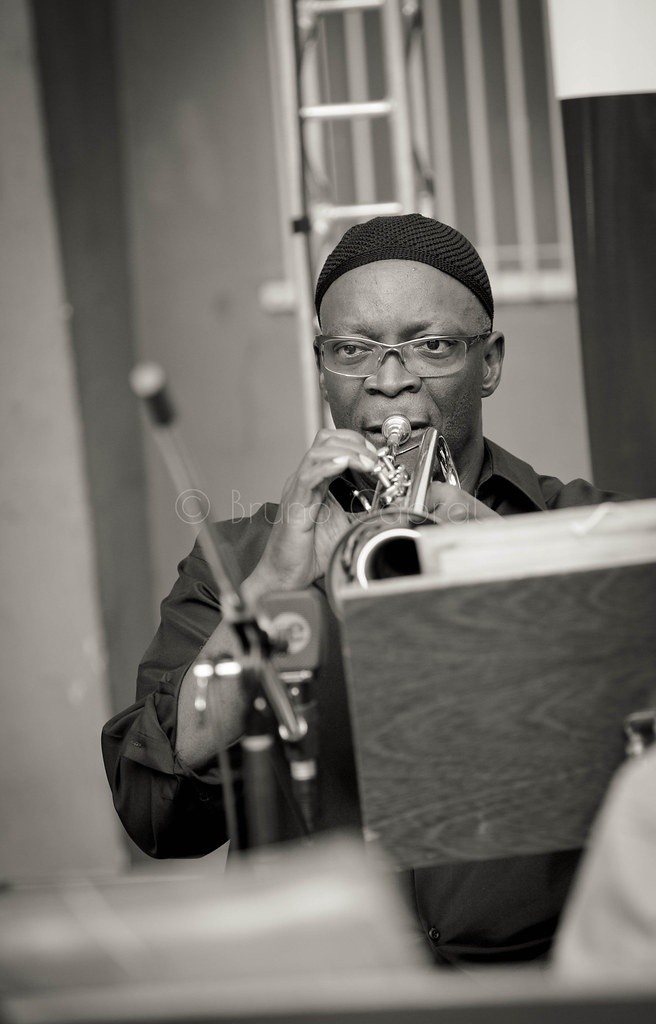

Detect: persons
[100, 211, 618, 865]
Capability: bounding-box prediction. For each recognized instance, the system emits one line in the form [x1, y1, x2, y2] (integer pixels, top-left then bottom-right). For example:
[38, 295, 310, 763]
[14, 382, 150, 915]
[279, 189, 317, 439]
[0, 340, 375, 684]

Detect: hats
[315, 213, 494, 330]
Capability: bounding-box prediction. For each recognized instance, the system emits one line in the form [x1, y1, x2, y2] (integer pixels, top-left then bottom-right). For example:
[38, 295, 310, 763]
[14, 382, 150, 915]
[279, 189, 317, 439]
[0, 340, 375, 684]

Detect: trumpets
[323, 411, 466, 629]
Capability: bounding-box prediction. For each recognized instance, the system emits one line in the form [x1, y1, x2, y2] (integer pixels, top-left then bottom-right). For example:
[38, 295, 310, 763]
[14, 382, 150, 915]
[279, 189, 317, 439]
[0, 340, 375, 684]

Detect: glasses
[313, 331, 491, 378]
